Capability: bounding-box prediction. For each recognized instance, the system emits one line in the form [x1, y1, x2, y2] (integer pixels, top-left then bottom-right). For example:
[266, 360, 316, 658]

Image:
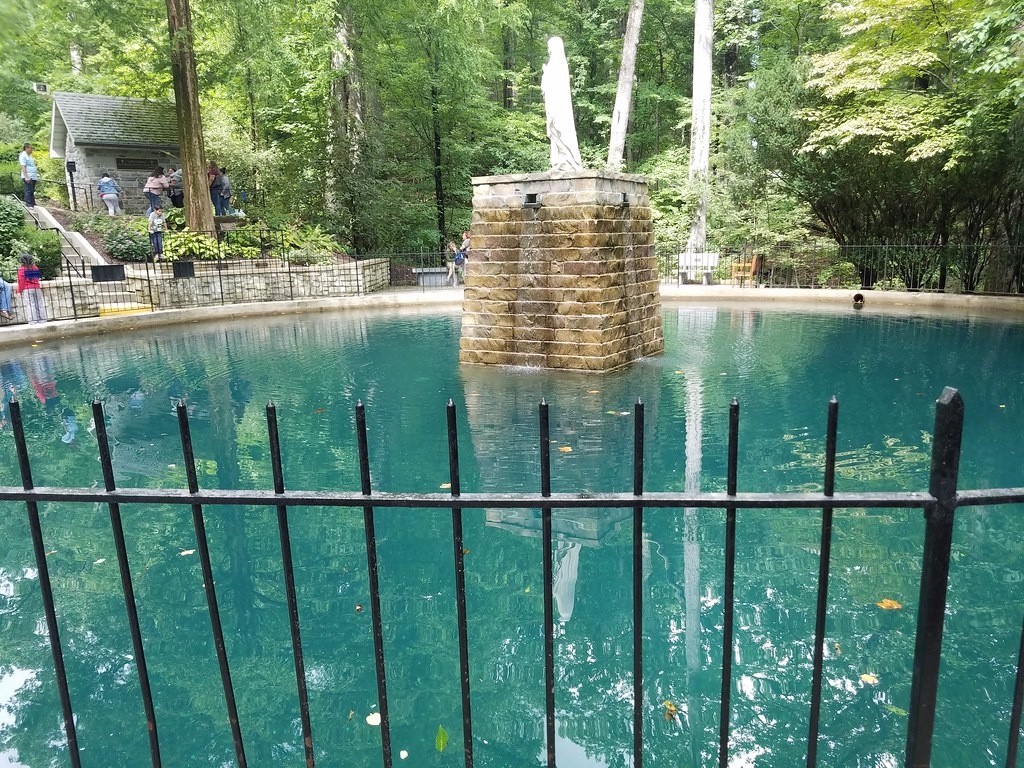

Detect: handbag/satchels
[455, 251, 465, 265]
[211, 174, 222, 187]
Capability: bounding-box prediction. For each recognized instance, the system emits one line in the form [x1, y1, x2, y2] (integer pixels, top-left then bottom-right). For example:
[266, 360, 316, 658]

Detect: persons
[458, 230, 470, 282]
[143, 165, 170, 218]
[205, 159, 234, 216]
[17, 254, 48, 325]
[0, 354, 79, 444]
[148, 205, 168, 260]
[19, 143, 38, 209]
[164, 167, 185, 208]
[0, 276, 15, 319]
[445, 241, 459, 287]
[98, 172, 127, 216]
[123, 381, 193, 418]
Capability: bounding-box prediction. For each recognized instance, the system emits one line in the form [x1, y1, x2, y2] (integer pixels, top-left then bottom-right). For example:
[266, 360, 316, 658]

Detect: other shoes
[153, 254, 159, 262]
[170, 193, 174, 197]
[446, 279, 450, 283]
[0, 310, 13, 320]
[453, 283, 457, 287]
[159, 254, 167, 259]
